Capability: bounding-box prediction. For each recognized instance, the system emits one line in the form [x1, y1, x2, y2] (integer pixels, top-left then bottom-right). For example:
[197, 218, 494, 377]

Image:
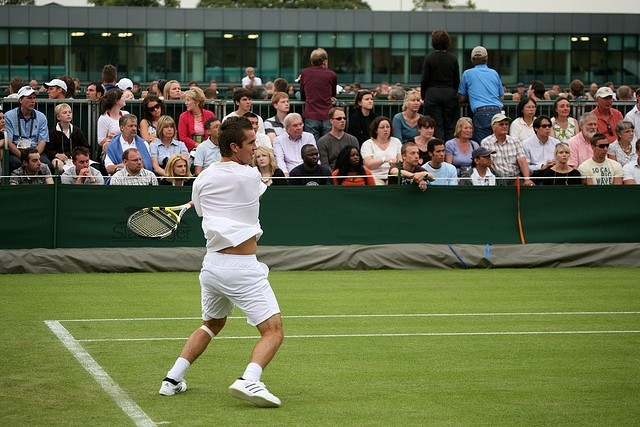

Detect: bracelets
[424, 172, 429, 179]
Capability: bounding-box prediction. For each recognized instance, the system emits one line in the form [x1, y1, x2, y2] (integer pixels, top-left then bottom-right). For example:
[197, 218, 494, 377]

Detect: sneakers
[228, 377, 281, 407]
[159, 378, 187, 396]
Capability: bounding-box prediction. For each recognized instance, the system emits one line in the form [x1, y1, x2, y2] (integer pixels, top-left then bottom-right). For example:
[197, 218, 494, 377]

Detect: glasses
[495, 123, 509, 126]
[594, 144, 609, 148]
[623, 131, 636, 135]
[332, 117, 347, 121]
[147, 104, 160, 112]
[542, 124, 552, 128]
[607, 124, 613, 136]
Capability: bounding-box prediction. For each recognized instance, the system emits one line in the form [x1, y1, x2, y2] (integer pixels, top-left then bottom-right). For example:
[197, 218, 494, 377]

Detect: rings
[530, 183, 534, 186]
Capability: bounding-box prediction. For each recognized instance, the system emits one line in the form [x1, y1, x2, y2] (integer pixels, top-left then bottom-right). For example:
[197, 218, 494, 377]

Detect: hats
[596, 87, 613, 98]
[43, 78, 67, 93]
[18, 86, 39, 99]
[491, 113, 510, 126]
[472, 46, 487, 57]
[471, 147, 496, 160]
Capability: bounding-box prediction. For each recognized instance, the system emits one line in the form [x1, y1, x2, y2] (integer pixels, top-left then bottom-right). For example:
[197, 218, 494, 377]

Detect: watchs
[90, 178, 98, 183]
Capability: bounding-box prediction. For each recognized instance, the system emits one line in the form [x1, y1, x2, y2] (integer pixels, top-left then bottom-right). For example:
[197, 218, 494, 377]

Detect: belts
[477, 107, 498, 112]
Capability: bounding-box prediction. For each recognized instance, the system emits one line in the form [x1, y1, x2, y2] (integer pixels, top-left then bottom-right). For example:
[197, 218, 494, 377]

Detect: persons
[524, 116, 561, 172]
[264, 92, 296, 139]
[543, 143, 581, 185]
[350, 91, 377, 140]
[140, 95, 178, 142]
[189, 82, 196, 89]
[4, 86, 50, 167]
[150, 115, 196, 180]
[360, 116, 403, 186]
[300, 48, 337, 141]
[289, 144, 331, 185]
[331, 145, 375, 186]
[253, 146, 288, 185]
[458, 45, 505, 147]
[163, 80, 181, 99]
[266, 81, 275, 92]
[421, 29, 460, 142]
[110, 148, 159, 186]
[30, 80, 38, 89]
[273, 113, 321, 181]
[157, 79, 167, 100]
[577, 134, 623, 185]
[481, 114, 536, 187]
[589, 87, 623, 144]
[43, 79, 68, 99]
[101, 64, 118, 91]
[9, 149, 54, 186]
[550, 97, 580, 140]
[60, 76, 75, 100]
[62, 147, 104, 185]
[567, 112, 599, 169]
[353, 83, 359, 92]
[388, 87, 406, 100]
[159, 116, 284, 408]
[9, 79, 22, 93]
[344, 84, 351, 93]
[149, 81, 158, 94]
[390, 142, 435, 192]
[104, 115, 153, 174]
[419, 139, 459, 186]
[97, 89, 135, 146]
[178, 88, 217, 151]
[622, 139, 640, 185]
[164, 154, 194, 186]
[608, 121, 637, 165]
[86, 82, 104, 100]
[317, 107, 360, 174]
[409, 117, 441, 161]
[460, 147, 497, 187]
[242, 67, 263, 88]
[222, 89, 264, 133]
[274, 78, 288, 92]
[445, 117, 479, 172]
[509, 98, 542, 144]
[512, 81, 640, 101]
[194, 117, 226, 176]
[623, 90, 640, 139]
[0, 110, 8, 181]
[287, 85, 295, 98]
[204, 80, 218, 99]
[242, 113, 272, 148]
[392, 91, 425, 141]
[416, 86, 422, 94]
[131, 81, 140, 92]
[47, 103, 89, 174]
